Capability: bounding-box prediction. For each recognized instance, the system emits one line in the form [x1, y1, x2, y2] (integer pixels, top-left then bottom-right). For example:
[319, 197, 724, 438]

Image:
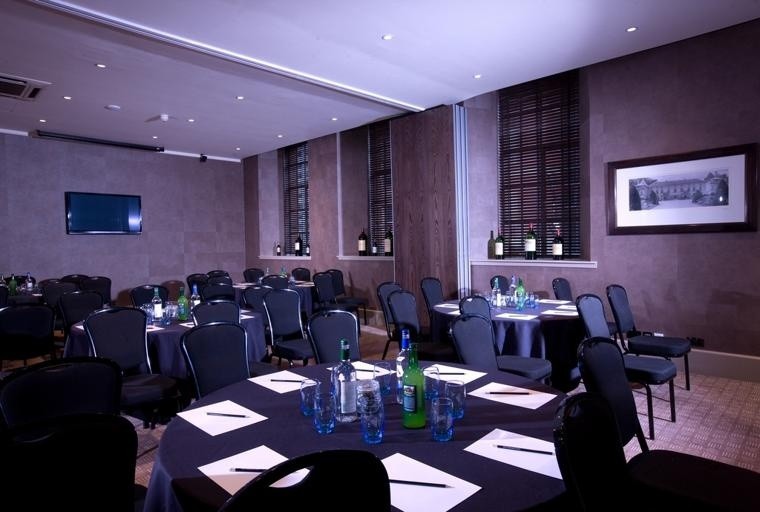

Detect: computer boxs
[200, 154, 207, 162]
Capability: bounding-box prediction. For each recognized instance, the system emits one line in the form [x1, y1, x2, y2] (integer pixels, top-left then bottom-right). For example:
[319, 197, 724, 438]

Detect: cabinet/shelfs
[275, 242, 281, 256]
[0, 272, 42, 297]
[265, 266, 288, 288]
[370, 240, 376, 255]
[494, 229, 505, 259]
[552, 229, 564, 259]
[304, 241, 310, 256]
[524, 222, 536, 259]
[357, 228, 369, 255]
[331, 330, 426, 429]
[491, 274, 525, 309]
[151, 285, 201, 321]
[294, 233, 303, 257]
[487, 230, 496, 259]
[383, 226, 393, 255]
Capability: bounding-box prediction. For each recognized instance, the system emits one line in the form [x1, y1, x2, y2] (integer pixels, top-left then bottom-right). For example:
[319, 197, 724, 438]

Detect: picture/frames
[605, 142, 760, 234]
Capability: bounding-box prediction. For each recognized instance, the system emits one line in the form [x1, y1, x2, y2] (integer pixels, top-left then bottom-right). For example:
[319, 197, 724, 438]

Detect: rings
[355, 368, 379, 372]
[230, 467, 266, 472]
[207, 412, 245, 418]
[485, 391, 532, 395]
[431, 373, 465, 375]
[390, 479, 453, 488]
[271, 379, 302, 382]
[497, 444, 554, 455]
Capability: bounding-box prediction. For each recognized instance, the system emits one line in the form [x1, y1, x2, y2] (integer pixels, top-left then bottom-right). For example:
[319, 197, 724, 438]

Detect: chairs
[607, 284, 691, 401]
[553, 391, 737, 511]
[577, 294, 678, 439]
[578, 337, 755, 505]
[0, 270, 622, 512]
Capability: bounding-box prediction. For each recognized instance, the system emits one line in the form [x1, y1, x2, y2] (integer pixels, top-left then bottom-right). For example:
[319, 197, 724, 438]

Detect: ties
[64, 191, 142, 235]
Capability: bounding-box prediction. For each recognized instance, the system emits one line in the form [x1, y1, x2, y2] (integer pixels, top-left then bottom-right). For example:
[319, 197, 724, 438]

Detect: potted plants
[605, 142, 760, 234]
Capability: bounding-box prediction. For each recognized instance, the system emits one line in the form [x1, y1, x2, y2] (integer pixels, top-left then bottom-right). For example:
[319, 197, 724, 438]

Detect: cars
[481, 288, 540, 311]
[299, 362, 466, 444]
[143, 301, 175, 325]
